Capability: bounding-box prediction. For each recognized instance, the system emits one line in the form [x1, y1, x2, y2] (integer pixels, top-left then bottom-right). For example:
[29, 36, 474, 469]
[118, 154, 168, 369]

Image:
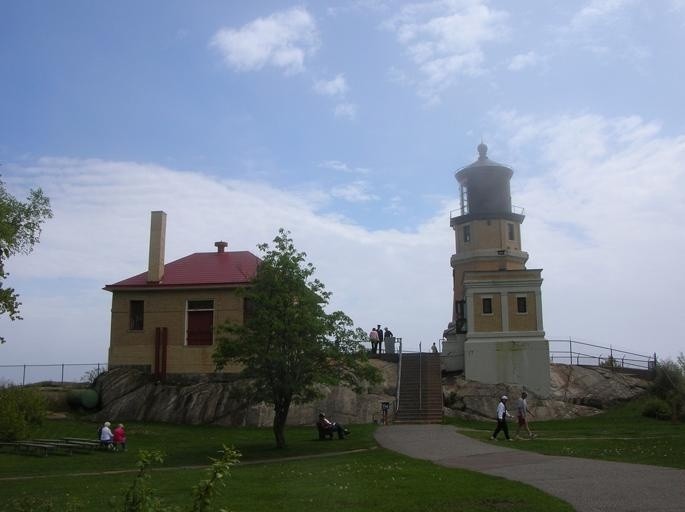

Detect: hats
[501, 396, 508, 400]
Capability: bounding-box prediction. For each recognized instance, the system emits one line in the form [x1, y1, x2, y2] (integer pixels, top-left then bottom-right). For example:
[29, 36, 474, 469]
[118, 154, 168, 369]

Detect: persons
[100, 422, 115, 450]
[376, 325, 383, 355]
[384, 327, 393, 341]
[370, 328, 380, 350]
[489, 395, 514, 442]
[112, 423, 128, 453]
[513, 391, 537, 441]
[431, 342, 438, 353]
[319, 411, 350, 440]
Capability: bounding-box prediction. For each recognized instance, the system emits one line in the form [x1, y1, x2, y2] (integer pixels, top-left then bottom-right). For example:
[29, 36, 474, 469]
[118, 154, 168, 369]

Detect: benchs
[316, 422, 333, 440]
[0, 437, 119, 457]
